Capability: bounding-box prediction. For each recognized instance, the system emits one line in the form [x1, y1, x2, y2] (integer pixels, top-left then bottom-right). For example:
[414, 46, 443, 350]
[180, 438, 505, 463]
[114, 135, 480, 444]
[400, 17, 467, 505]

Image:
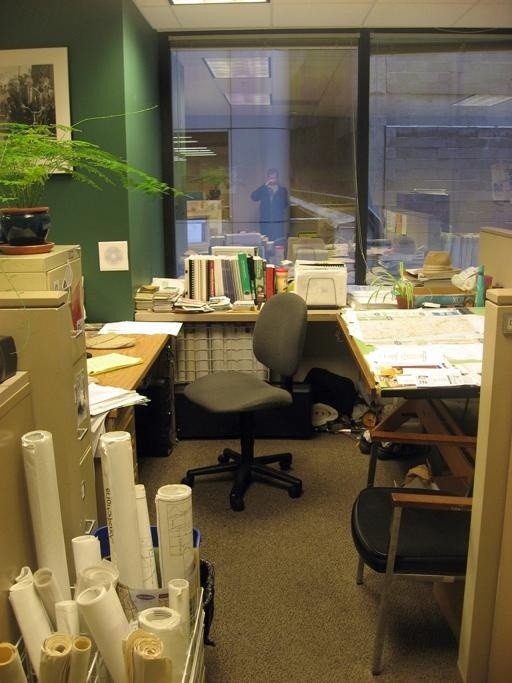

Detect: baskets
[16, 585, 206, 682]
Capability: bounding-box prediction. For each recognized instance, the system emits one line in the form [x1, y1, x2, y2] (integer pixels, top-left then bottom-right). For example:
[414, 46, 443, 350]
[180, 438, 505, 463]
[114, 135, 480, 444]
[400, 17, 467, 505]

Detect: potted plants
[185, 159, 243, 199]
[0, 91, 193, 259]
[362, 258, 433, 311]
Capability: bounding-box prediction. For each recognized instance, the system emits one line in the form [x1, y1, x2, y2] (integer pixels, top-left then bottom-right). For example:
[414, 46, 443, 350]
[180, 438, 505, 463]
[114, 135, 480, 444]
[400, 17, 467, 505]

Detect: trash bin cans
[199, 559, 214, 640]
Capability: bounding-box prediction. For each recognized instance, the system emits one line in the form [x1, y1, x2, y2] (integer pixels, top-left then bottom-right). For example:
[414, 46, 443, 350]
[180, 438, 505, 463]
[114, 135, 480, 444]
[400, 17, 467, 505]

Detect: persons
[250, 169, 287, 241]
[7, 72, 56, 123]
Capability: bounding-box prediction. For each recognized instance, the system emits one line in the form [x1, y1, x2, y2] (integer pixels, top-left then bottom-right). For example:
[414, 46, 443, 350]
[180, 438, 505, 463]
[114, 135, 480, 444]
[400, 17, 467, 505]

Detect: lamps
[449, 258, 491, 311]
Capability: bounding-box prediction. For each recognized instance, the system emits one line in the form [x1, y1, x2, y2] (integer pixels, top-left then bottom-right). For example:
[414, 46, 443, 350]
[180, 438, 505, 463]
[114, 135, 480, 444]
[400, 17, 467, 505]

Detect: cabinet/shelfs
[0, 241, 102, 603]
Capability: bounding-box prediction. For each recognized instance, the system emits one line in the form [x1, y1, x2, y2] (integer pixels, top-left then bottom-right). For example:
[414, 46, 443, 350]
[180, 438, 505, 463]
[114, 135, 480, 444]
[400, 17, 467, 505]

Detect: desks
[334, 299, 492, 499]
[79, 319, 183, 534]
[125, 272, 399, 441]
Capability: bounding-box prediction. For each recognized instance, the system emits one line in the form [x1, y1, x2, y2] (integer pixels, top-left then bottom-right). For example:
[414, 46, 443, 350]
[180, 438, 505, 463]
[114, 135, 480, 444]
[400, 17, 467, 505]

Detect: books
[134, 245, 276, 311]
[346, 268, 476, 310]
[444, 232, 479, 269]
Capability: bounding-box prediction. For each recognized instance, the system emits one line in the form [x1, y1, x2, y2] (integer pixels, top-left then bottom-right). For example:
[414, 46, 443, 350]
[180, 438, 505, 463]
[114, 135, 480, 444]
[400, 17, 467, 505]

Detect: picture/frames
[0, 44, 76, 177]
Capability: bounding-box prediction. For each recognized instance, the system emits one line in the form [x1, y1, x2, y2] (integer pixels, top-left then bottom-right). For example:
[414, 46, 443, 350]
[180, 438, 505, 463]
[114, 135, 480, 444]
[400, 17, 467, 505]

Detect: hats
[406, 251, 462, 275]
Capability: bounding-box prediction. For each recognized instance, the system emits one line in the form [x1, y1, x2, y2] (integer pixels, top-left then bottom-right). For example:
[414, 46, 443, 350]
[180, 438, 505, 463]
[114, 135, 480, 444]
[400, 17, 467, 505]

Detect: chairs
[347, 426, 484, 679]
[177, 288, 309, 514]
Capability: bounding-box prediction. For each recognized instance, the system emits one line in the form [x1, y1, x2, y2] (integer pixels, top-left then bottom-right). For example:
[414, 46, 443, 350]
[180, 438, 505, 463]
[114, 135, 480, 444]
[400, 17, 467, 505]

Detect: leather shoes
[360, 434, 419, 460]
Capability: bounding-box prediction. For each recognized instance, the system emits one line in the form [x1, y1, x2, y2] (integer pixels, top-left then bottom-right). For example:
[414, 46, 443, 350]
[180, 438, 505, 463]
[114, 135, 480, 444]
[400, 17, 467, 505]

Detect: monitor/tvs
[187, 215, 209, 250]
